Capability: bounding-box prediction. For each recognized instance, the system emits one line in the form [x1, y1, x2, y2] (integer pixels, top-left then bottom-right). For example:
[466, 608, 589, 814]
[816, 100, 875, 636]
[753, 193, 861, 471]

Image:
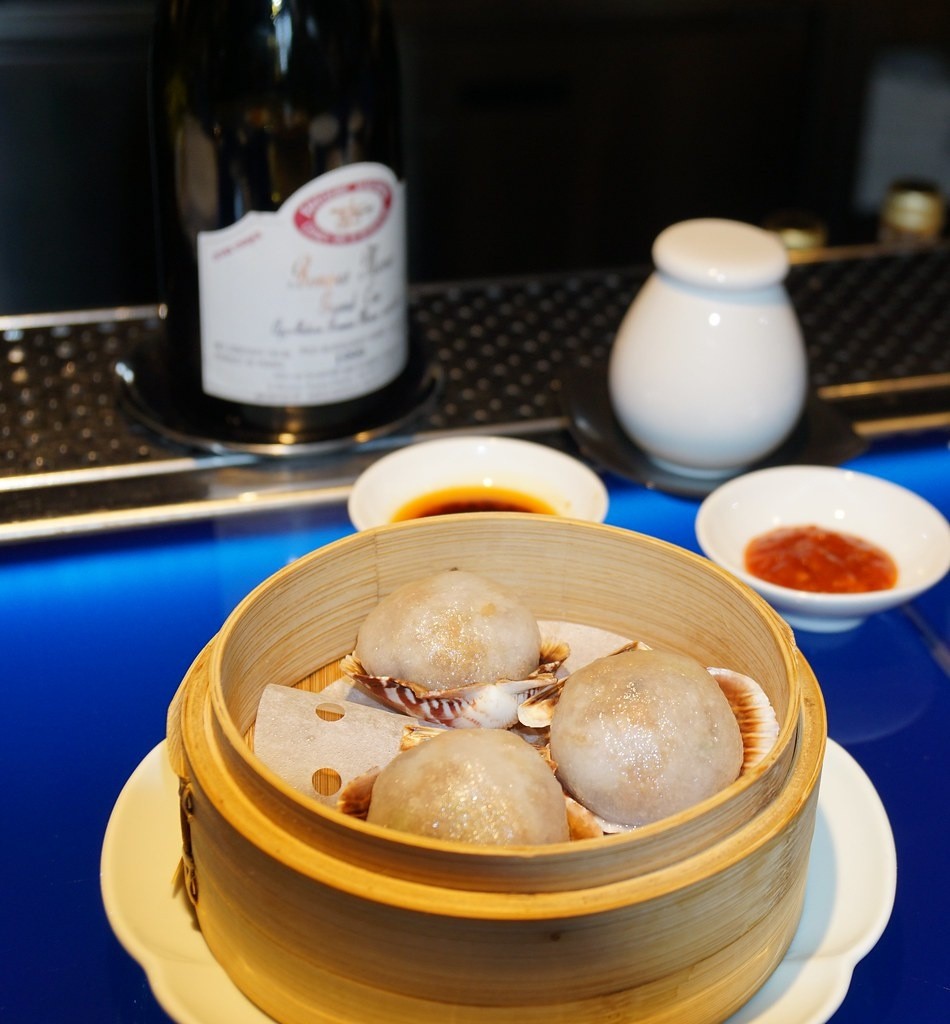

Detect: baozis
[365, 728, 571, 845]
[356, 570, 541, 691]
[549, 650, 744, 826]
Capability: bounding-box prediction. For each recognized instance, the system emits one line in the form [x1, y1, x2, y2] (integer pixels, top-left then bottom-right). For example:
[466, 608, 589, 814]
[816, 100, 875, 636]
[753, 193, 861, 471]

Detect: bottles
[607, 219, 807, 480]
[166, 0, 423, 440]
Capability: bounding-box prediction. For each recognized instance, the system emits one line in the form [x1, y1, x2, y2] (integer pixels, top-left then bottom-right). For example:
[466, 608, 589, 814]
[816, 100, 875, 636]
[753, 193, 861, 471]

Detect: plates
[107, 314, 445, 458]
[98, 739, 898, 1022]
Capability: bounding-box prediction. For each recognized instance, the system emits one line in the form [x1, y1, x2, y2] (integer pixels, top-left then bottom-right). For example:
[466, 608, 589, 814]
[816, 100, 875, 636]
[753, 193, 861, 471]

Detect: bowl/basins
[694, 466, 950, 636]
[347, 436, 609, 532]
